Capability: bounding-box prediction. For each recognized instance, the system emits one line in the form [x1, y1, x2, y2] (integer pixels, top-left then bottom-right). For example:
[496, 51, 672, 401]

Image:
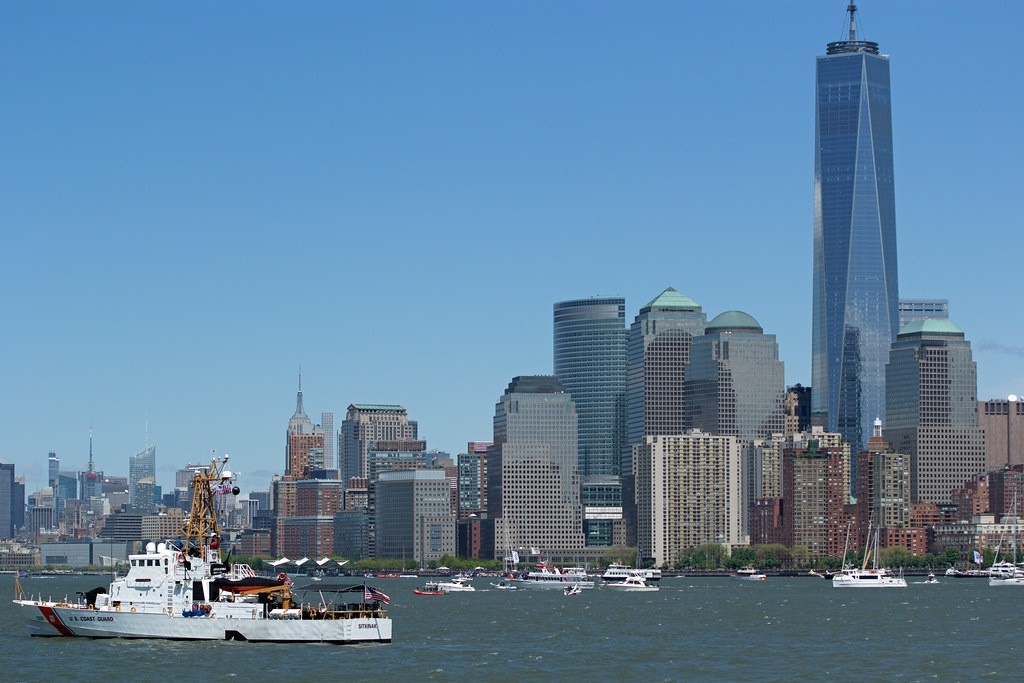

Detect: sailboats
[988, 487, 1024, 586]
[808, 519, 908, 588]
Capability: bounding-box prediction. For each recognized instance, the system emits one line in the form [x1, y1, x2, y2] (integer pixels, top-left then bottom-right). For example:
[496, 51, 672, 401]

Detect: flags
[216, 484, 231, 495]
[364, 586, 390, 605]
[512, 551, 519, 564]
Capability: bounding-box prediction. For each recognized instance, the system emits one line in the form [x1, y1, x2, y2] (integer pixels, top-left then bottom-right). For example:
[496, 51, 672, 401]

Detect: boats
[11, 447, 392, 645]
[606, 571, 660, 592]
[736, 565, 767, 581]
[505, 551, 595, 590]
[945, 557, 1024, 578]
[413, 572, 476, 596]
[924, 572, 939, 584]
[563, 584, 581, 596]
[601, 560, 662, 581]
[490, 583, 518, 590]
[212, 576, 292, 592]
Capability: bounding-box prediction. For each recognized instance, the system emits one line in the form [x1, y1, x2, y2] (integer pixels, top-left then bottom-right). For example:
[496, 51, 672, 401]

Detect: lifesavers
[279, 573, 286, 580]
[42, 601, 47, 607]
[88, 604, 94, 610]
[131, 607, 136, 611]
[116, 605, 121, 611]
